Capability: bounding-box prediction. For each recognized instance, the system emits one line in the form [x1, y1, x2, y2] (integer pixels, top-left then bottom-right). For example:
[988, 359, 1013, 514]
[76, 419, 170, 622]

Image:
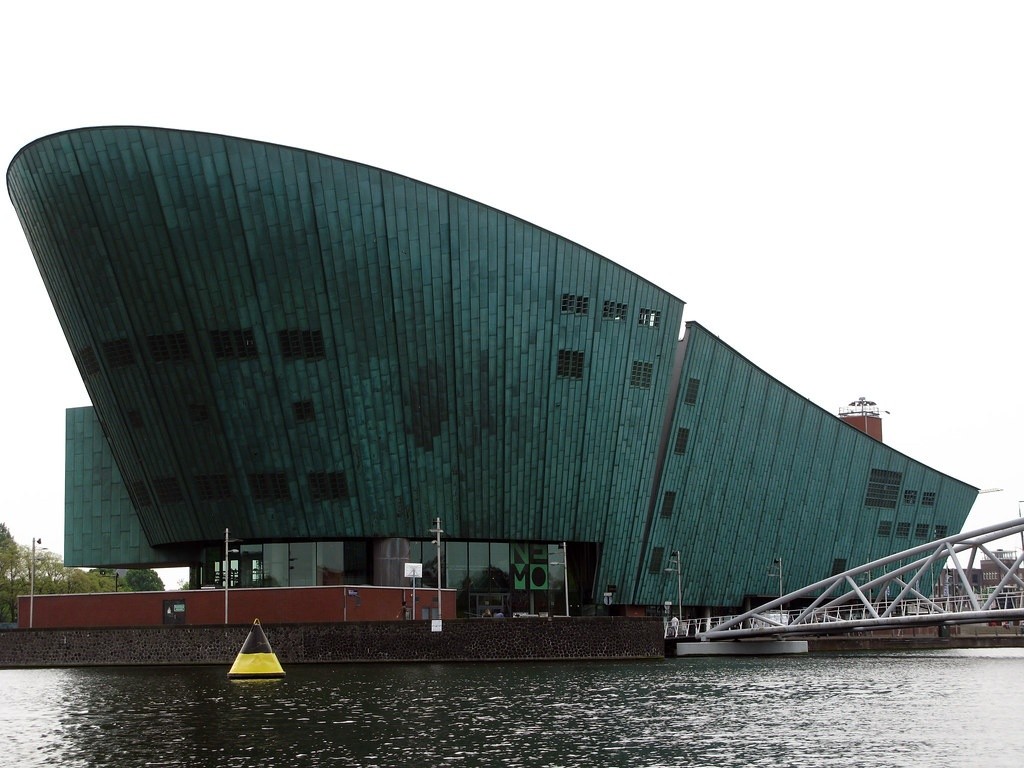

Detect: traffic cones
[226, 620, 290, 679]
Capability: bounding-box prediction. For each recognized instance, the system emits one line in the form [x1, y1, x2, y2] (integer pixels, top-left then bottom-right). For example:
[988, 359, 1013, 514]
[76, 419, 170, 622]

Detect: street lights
[859, 556, 903, 619]
[661, 551, 683, 620]
[549, 542, 571, 619]
[430, 517, 444, 620]
[768, 557, 784, 627]
[223, 525, 239, 623]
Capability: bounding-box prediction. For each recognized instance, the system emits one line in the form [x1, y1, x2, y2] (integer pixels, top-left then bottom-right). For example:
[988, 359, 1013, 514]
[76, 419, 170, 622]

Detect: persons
[670, 615, 679, 636]
[481, 609, 504, 618]
[397, 601, 408, 620]
[991, 621, 1011, 629]
